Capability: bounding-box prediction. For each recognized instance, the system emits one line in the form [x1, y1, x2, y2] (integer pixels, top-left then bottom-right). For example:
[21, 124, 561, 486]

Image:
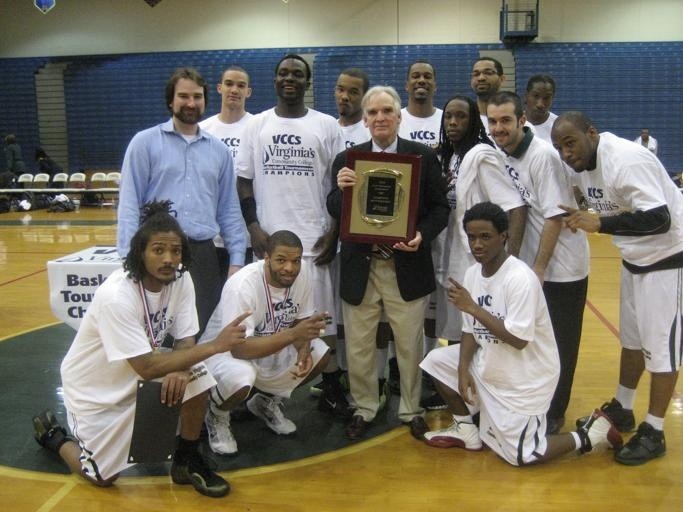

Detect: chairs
[0, 171, 123, 207]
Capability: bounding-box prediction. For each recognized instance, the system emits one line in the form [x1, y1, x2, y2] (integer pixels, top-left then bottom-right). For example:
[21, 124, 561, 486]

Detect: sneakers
[171, 391, 297, 497]
[309, 366, 482, 451]
[33, 409, 67, 447]
[546, 397, 666, 466]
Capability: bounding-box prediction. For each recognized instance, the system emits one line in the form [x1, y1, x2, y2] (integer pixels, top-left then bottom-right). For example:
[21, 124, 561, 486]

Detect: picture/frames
[338, 151, 421, 247]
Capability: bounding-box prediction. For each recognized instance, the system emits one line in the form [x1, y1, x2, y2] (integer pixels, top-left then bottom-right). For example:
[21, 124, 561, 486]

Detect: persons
[422, 200, 625, 469]
[2, 134, 26, 176]
[633, 129, 659, 156]
[30, 197, 231, 500]
[116, 55, 591, 440]
[192, 227, 331, 455]
[548, 110, 680, 466]
[43, 193, 75, 212]
